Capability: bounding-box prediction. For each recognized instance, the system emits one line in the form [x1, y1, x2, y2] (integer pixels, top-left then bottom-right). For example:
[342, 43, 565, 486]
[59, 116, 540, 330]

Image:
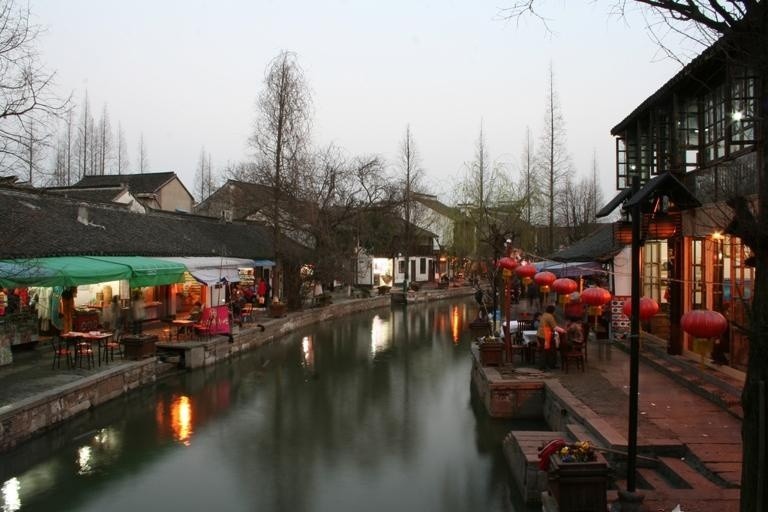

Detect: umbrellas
[545, 261, 603, 293]
[532, 260, 560, 273]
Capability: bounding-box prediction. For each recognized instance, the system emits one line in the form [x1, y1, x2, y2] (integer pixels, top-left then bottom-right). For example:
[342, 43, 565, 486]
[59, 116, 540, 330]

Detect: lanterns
[516, 265, 537, 286]
[681, 307, 728, 356]
[551, 278, 577, 304]
[622, 296, 659, 321]
[578, 287, 612, 318]
[535, 271, 556, 293]
[499, 257, 517, 278]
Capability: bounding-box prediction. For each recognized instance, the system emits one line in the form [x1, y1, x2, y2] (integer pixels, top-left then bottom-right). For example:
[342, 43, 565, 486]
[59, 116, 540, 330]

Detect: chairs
[49, 328, 125, 370]
[193, 312, 214, 342]
[502, 319, 590, 374]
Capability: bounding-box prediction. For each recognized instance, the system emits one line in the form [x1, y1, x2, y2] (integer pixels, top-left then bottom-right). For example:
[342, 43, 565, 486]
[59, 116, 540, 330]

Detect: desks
[169, 320, 194, 341]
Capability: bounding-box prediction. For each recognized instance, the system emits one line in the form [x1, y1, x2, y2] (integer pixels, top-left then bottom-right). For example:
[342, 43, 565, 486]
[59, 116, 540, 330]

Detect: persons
[177, 301, 202, 336]
[110, 294, 123, 335]
[131, 294, 144, 334]
[256, 276, 267, 308]
[537, 304, 567, 371]
[532, 311, 543, 330]
[510, 247, 532, 266]
[565, 317, 584, 353]
